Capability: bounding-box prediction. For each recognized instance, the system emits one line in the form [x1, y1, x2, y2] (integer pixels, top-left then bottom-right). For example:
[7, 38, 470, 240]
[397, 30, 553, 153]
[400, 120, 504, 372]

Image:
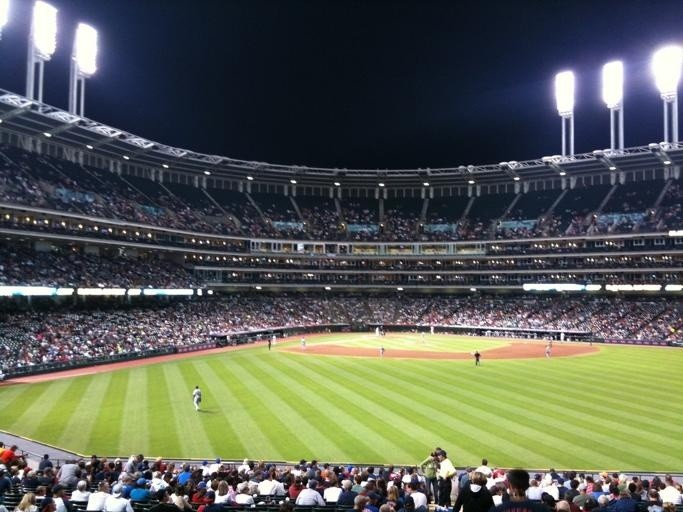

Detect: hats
[51, 485, 66, 494]
[112, 484, 122, 498]
[401, 473, 419, 484]
[340, 480, 352, 490]
[202, 492, 214, 503]
[136, 478, 148, 486]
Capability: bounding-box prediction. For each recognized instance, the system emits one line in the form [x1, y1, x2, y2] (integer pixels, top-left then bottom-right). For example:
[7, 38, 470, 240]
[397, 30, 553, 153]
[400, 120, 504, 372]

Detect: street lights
[553, 70, 575, 158]
[0, 0, 11, 38]
[24, 0, 60, 112]
[600, 60, 624, 154]
[68, 20, 99, 116]
[650, 43, 683, 148]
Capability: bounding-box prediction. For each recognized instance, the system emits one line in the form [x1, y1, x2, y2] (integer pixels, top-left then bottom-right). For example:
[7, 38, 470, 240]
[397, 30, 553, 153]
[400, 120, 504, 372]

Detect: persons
[544, 345, 551, 359]
[267, 340, 271, 350]
[589, 340, 592, 347]
[300, 338, 306, 351]
[379, 346, 384, 357]
[0, 441, 683, 511]
[474, 352, 481, 365]
[1, 153, 265, 379]
[267, 198, 683, 345]
[192, 386, 201, 410]
